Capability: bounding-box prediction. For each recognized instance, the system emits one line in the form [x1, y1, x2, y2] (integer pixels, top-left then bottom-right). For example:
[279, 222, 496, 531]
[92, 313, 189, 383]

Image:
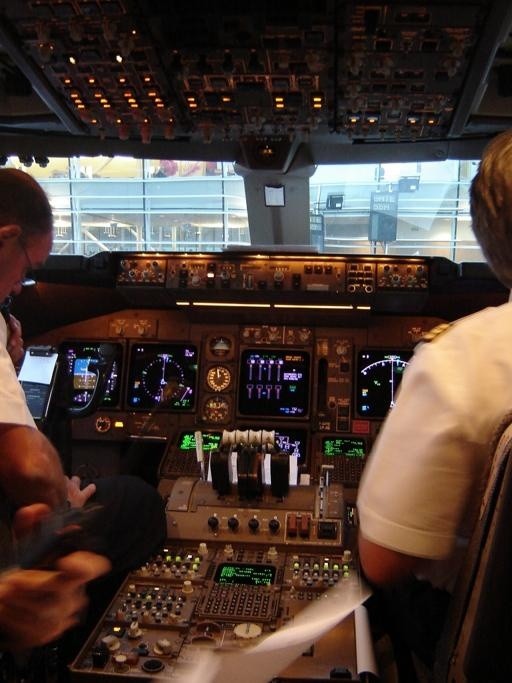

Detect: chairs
[407, 404, 511, 682]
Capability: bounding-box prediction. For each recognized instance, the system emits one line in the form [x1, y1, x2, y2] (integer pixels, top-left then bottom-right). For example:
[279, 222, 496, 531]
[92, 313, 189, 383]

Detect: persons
[1, 161, 95, 534]
[1, 501, 112, 654]
[354, 129, 512, 611]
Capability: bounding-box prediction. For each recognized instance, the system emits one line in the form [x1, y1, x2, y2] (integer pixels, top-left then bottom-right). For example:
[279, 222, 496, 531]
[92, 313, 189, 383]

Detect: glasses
[15, 235, 38, 289]
